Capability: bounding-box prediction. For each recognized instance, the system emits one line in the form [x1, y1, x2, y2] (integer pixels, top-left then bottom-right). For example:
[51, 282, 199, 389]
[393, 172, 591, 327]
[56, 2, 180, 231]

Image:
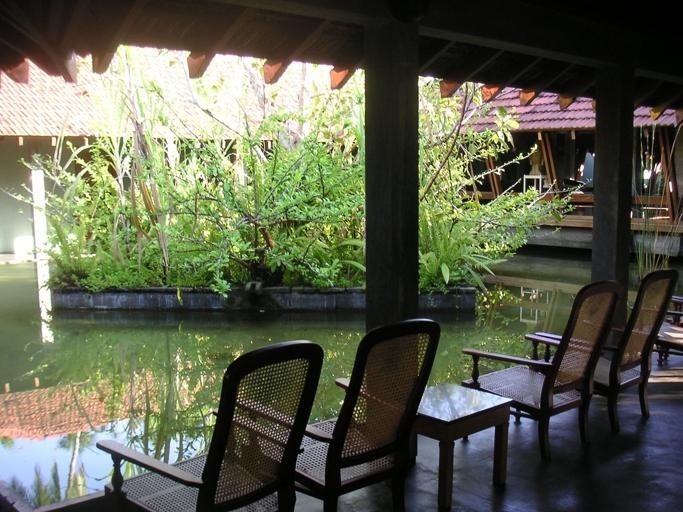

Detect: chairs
[96, 339, 324, 512]
[233, 317, 442, 511]
[533, 270, 681, 435]
[461, 280, 625, 471]
[630, 296, 683, 368]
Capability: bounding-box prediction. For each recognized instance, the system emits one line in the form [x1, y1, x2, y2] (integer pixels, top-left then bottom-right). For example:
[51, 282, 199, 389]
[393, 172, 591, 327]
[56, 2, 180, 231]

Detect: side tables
[415, 382, 514, 510]
